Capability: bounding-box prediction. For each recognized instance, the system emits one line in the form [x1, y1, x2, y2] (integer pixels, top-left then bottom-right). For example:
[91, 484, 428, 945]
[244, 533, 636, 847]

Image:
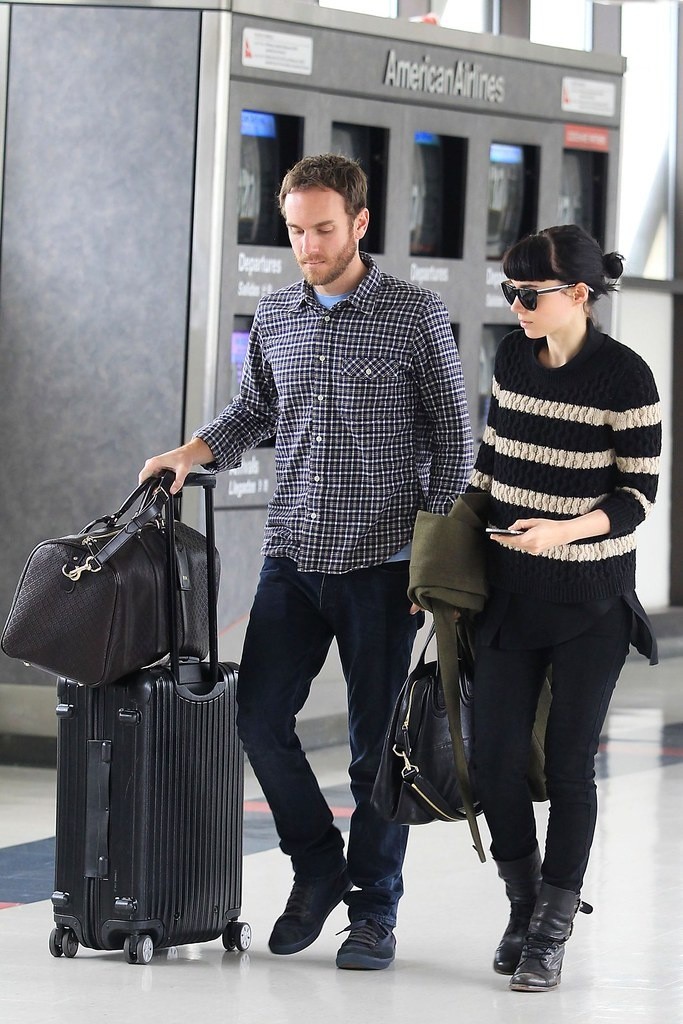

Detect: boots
[509, 879, 593, 992]
[490, 839, 543, 975]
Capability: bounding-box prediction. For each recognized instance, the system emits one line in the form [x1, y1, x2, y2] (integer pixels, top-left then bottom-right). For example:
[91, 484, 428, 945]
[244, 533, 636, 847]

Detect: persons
[465, 225, 662, 992]
[139, 161, 475, 971]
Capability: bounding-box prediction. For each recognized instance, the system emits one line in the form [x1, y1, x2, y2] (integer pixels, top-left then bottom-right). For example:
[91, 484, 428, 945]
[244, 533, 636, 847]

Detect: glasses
[501, 280, 594, 311]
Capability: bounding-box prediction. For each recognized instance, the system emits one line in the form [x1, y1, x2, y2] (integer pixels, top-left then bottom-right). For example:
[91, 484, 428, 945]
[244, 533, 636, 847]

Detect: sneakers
[336, 917, 396, 970]
[268, 857, 354, 955]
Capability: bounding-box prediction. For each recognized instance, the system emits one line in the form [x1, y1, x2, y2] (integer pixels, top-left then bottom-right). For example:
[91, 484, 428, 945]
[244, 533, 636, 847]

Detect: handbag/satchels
[0, 476, 221, 688]
[369, 621, 552, 825]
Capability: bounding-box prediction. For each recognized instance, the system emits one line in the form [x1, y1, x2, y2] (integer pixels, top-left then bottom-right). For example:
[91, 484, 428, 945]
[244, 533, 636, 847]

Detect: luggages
[48, 473, 252, 964]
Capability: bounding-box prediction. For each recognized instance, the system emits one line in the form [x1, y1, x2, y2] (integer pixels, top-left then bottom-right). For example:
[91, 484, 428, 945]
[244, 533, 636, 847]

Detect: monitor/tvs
[229, 109, 604, 447]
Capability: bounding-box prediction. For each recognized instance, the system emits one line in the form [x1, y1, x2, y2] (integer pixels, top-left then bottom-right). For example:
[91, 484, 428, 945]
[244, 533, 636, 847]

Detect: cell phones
[486, 528, 524, 536]
